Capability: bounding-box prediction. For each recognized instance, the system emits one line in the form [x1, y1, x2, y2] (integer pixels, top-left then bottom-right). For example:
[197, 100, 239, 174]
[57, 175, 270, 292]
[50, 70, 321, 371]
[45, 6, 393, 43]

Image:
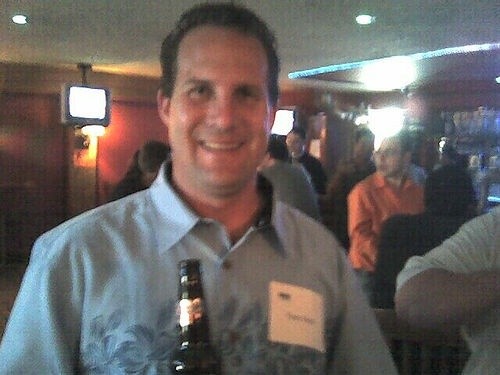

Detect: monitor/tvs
[60, 82, 111, 127]
[270, 104, 298, 136]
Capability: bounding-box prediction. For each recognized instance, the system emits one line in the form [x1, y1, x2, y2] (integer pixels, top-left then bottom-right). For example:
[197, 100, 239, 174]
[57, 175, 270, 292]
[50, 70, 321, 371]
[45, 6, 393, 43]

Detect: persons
[394, 195, 500, 374]
[255, 133, 322, 225]
[373, 161, 483, 319]
[343, 135, 435, 286]
[329, 126, 384, 255]
[278, 130, 329, 200]
[109, 140, 174, 208]
[0, 0, 401, 375]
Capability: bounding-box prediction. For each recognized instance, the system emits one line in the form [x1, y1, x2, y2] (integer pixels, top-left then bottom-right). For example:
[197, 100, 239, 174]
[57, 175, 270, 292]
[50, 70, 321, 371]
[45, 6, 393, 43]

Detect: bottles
[171, 258, 222, 375]
